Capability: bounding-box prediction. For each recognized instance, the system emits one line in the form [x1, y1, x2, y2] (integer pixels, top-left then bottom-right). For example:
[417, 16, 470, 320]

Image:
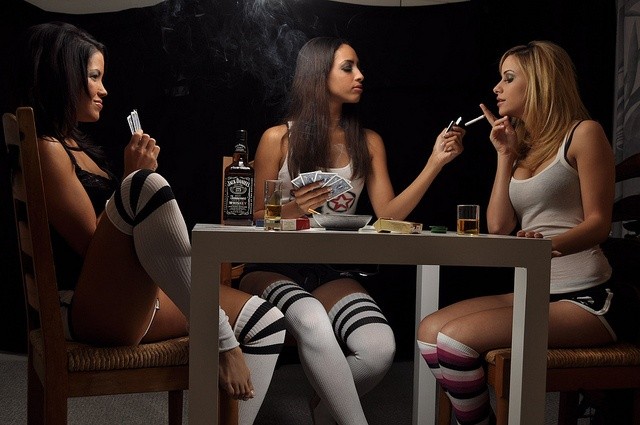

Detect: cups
[264, 180, 284, 232]
[457, 204, 480, 236]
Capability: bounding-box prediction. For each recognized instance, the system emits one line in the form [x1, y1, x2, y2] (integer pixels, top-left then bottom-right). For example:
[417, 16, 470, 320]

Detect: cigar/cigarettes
[308, 208, 320, 214]
[465, 114, 486, 126]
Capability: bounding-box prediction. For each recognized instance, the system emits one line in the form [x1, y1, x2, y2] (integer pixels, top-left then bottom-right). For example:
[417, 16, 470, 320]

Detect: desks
[190, 223, 554, 425]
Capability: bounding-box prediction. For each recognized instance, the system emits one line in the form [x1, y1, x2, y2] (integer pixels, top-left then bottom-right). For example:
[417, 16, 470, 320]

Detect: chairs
[1, 106, 239, 425]
[434, 152, 640, 425]
[222, 155, 295, 347]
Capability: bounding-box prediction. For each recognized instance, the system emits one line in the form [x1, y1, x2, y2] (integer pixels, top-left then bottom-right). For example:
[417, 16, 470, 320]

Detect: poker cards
[291, 170, 354, 202]
[126, 108, 141, 136]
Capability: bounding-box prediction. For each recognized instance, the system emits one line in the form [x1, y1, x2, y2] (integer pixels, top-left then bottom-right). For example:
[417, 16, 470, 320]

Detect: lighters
[446, 117, 462, 133]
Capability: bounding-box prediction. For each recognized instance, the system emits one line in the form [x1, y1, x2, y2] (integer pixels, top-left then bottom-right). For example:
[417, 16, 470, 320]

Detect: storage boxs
[375, 217, 423, 233]
[280, 217, 311, 231]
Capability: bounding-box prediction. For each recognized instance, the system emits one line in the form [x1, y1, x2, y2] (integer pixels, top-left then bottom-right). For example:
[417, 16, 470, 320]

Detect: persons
[418, 41, 615, 425]
[15, 20, 287, 425]
[239, 36, 466, 425]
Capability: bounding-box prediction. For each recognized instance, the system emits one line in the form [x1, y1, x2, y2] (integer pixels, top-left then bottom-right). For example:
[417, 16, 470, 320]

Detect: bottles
[222, 129, 254, 227]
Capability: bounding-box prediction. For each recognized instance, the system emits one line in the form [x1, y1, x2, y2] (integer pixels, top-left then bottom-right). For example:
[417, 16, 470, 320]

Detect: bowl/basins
[312, 213, 373, 231]
